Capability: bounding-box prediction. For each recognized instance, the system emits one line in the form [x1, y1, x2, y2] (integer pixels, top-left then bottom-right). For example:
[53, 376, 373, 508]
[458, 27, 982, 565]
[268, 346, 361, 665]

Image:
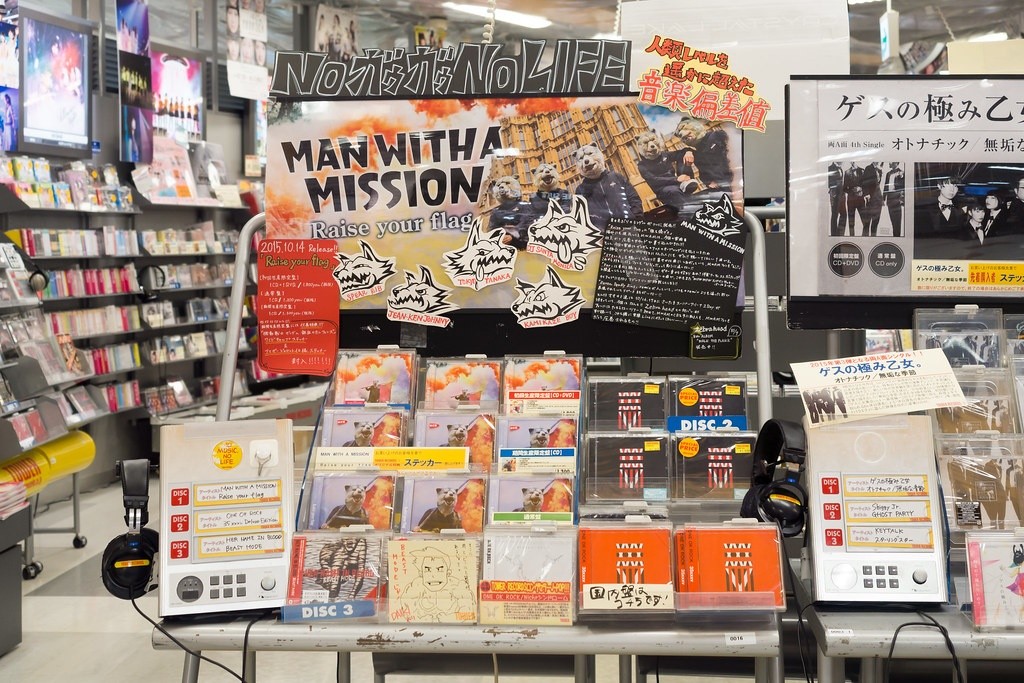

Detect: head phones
[137, 264, 165, 301]
[13, 244, 50, 291]
[103, 457, 159, 601]
[737, 418, 808, 538]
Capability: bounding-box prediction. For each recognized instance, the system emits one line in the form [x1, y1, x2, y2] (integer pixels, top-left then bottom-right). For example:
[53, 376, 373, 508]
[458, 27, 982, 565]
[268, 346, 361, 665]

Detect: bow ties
[941, 204, 954, 211]
[984, 216, 993, 220]
[976, 225, 983, 231]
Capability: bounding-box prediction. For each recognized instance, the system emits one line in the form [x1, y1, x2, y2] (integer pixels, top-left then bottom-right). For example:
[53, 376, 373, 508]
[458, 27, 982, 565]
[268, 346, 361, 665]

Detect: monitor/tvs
[15, 5, 93, 160]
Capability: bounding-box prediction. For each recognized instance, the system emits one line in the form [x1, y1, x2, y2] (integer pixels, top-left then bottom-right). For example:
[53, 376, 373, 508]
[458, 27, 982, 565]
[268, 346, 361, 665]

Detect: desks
[783, 560, 1022, 683]
[150, 608, 781, 683]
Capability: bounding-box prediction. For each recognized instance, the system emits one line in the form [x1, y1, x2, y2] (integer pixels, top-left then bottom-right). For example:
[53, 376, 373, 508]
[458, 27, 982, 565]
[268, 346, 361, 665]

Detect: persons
[925, 327, 1024, 531]
[914, 172, 1024, 261]
[0, 26, 20, 88]
[488, 115, 733, 251]
[156, 92, 201, 139]
[828, 161, 905, 237]
[2, 94, 17, 152]
[804, 387, 847, 423]
[317, 15, 357, 60]
[118, 16, 148, 162]
[225, 0, 268, 67]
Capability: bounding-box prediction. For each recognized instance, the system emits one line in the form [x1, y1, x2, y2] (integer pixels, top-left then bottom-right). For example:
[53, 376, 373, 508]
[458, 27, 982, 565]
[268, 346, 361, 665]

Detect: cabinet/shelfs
[0, 183, 315, 420]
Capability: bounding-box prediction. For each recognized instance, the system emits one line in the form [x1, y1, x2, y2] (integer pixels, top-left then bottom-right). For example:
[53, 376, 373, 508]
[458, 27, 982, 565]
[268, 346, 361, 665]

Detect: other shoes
[680, 179, 698, 194]
